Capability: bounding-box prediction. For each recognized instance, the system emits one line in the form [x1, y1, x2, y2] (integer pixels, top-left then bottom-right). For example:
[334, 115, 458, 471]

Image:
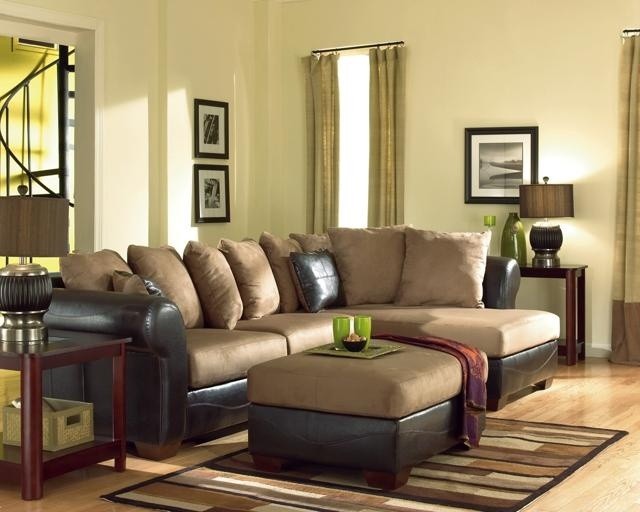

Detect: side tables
[0, 330, 132, 501]
[520, 265, 588, 366]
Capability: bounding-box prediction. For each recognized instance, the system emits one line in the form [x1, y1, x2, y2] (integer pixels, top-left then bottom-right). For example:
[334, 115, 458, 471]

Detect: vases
[501, 212, 527, 266]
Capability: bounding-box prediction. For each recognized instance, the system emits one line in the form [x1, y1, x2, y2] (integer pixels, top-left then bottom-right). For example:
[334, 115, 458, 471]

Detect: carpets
[100, 417, 629, 512]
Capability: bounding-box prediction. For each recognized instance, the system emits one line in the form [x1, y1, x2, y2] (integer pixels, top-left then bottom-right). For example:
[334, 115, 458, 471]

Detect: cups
[333, 315, 371, 350]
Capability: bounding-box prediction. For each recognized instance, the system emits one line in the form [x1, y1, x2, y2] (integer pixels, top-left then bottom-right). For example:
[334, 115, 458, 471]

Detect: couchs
[45, 255, 560, 461]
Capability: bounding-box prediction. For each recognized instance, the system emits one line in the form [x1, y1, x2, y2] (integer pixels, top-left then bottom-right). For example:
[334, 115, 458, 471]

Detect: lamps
[1, 184, 69, 342]
[519, 177, 574, 267]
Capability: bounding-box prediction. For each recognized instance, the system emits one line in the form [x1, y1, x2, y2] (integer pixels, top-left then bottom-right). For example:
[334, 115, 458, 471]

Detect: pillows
[59, 248, 133, 295]
[292, 248, 348, 313]
[185, 240, 245, 331]
[219, 236, 281, 321]
[262, 230, 307, 314]
[291, 232, 332, 253]
[328, 227, 405, 306]
[401, 226, 492, 309]
[129, 242, 203, 329]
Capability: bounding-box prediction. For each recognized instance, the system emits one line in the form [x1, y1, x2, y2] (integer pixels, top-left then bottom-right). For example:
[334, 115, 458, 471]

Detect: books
[12, 396, 56, 413]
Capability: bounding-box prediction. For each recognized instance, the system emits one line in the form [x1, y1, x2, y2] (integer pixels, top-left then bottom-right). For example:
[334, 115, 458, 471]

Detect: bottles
[484, 215, 498, 256]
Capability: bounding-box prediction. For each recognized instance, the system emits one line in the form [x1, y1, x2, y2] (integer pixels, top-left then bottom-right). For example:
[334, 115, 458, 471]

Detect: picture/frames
[195, 99, 228, 160]
[194, 164, 230, 224]
[463, 126, 538, 203]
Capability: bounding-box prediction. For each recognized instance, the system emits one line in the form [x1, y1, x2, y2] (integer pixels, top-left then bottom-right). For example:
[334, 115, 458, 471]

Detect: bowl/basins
[342, 336, 367, 352]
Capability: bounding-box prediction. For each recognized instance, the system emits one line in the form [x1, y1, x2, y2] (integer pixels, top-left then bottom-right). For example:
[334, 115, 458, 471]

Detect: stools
[247, 337, 489, 491]
